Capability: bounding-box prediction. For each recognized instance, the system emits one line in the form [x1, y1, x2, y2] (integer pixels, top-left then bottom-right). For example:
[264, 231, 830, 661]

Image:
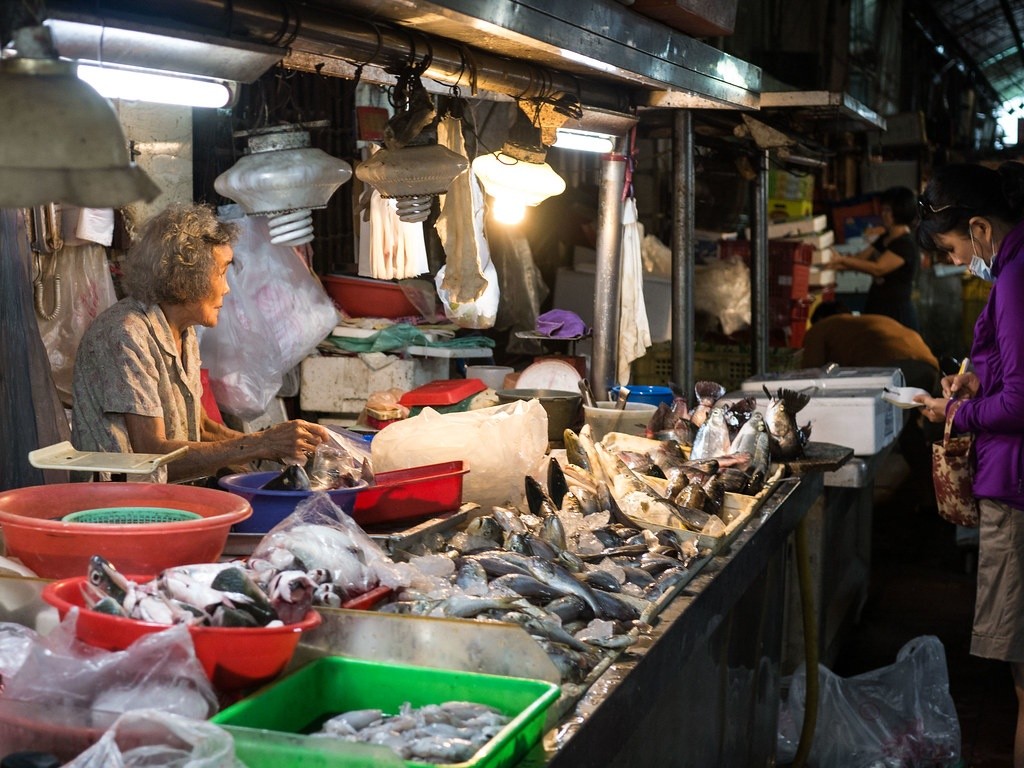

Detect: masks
[968, 253, 997, 285]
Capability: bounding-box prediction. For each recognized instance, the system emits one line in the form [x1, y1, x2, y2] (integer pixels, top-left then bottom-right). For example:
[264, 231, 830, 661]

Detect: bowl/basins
[40, 579, 323, 690]
[208, 655, 563, 767]
[218, 470, 370, 534]
[346, 458, 475, 526]
[0, 480, 254, 580]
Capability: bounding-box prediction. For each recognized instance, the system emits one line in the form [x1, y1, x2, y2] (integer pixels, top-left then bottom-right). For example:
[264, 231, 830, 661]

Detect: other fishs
[81, 381, 812, 683]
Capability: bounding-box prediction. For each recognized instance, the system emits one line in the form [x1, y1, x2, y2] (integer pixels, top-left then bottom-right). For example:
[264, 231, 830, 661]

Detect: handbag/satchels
[930, 398, 980, 529]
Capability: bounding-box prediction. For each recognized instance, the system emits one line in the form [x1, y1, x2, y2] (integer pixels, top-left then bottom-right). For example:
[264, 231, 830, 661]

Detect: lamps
[68, 56, 241, 114]
[469, 100, 564, 228]
[215, 65, 349, 248]
[356, 54, 468, 222]
[552, 127, 619, 156]
[0, 0, 160, 208]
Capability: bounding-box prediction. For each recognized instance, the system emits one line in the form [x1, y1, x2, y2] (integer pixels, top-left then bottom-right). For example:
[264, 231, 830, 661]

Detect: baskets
[60, 505, 204, 531]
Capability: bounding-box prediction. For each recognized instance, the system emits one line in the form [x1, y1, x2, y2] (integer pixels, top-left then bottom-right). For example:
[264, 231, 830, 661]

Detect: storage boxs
[745, 370, 906, 453]
[630, 340, 803, 389]
[213, 655, 558, 767]
[225, 399, 294, 475]
[351, 460, 470, 527]
[694, 170, 835, 349]
[300, 328, 493, 412]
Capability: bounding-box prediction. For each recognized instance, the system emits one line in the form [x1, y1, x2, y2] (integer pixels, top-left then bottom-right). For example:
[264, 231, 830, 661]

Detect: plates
[398, 378, 486, 408]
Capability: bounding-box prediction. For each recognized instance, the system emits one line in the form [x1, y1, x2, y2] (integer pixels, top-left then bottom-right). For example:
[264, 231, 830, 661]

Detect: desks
[528, 447, 854, 767]
[826, 449, 908, 641]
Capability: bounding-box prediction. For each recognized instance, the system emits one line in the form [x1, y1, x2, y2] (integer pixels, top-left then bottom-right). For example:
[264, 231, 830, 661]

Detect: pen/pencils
[949, 358, 971, 401]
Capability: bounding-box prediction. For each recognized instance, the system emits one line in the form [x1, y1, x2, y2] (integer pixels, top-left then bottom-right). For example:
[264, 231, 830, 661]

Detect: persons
[799, 298, 946, 456]
[913, 159, 1023, 767]
[826, 182, 923, 325]
[63, 206, 329, 488]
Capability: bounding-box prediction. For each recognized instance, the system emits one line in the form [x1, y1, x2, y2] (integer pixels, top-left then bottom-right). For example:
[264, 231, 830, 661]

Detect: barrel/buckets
[496, 390, 584, 441]
[613, 386, 673, 407]
[582, 401, 659, 448]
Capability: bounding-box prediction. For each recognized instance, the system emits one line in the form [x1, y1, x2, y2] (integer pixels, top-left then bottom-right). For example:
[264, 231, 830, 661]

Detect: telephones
[30, 202, 64, 255]
